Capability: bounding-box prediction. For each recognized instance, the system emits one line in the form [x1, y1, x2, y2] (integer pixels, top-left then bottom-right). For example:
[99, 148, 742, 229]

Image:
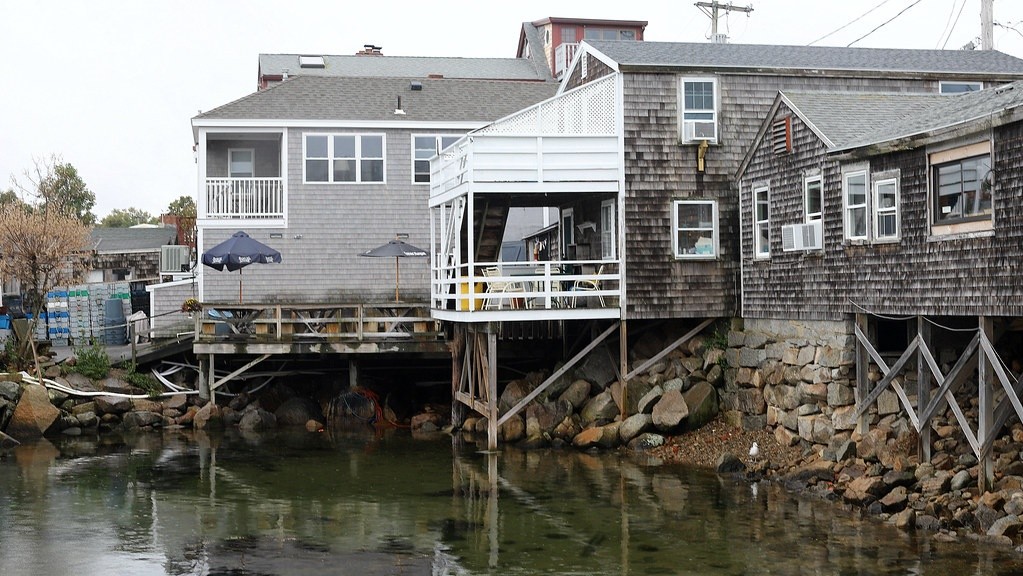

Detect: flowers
[181, 299, 203, 313]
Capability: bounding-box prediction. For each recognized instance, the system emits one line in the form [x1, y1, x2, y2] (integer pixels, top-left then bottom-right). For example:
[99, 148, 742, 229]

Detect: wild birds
[749, 442, 760, 464]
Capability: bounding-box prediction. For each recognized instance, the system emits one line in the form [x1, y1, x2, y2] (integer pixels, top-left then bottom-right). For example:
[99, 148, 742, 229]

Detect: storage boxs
[0, 315, 11, 329]
[23, 283, 132, 347]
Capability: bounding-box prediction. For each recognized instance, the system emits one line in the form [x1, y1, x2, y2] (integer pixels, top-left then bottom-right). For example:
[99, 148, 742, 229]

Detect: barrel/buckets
[104, 299, 127, 345]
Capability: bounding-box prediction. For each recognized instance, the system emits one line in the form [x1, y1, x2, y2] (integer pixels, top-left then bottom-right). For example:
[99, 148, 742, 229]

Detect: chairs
[12, 318, 51, 353]
[481, 265, 605, 310]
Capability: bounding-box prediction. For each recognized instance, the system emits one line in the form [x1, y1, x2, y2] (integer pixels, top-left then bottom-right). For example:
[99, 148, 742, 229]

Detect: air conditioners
[782, 224, 816, 252]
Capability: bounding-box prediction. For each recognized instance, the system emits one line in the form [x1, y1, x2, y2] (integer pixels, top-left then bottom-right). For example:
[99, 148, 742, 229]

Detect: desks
[510, 274, 570, 309]
[217, 304, 265, 338]
[374, 301, 418, 340]
[290, 304, 339, 341]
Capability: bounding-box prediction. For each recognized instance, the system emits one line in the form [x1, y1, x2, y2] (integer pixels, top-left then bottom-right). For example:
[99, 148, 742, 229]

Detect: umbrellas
[202, 231, 282, 319]
[357, 237, 430, 316]
